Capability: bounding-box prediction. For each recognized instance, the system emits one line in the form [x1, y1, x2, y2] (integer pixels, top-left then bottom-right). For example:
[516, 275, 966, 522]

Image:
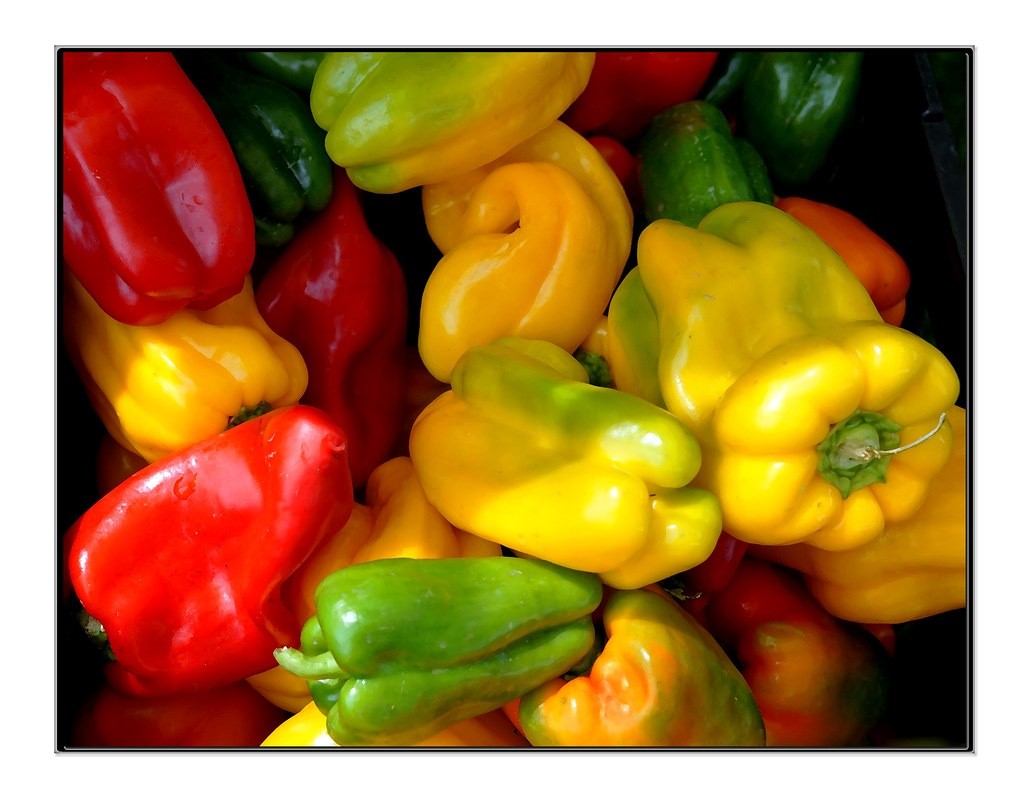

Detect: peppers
[57, 45, 970, 747]
